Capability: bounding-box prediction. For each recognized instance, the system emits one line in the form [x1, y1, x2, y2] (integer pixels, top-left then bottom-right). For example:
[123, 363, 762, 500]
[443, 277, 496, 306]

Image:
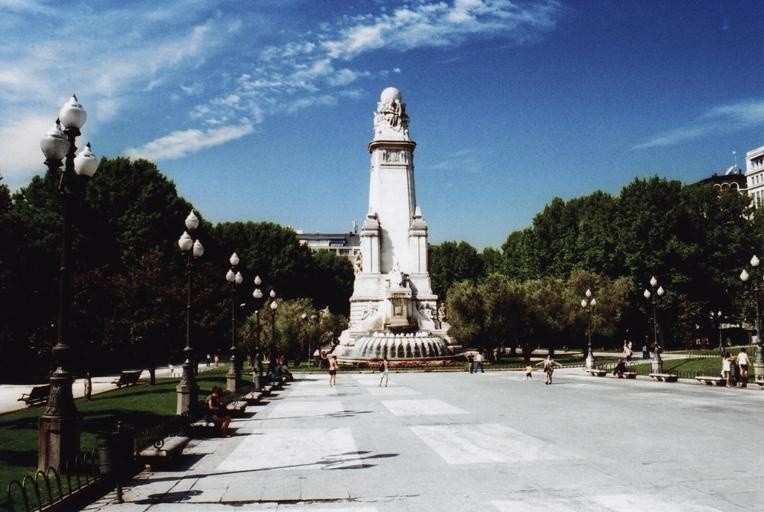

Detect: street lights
[225, 251, 243, 393]
[37, 92, 98, 478]
[269, 289, 278, 378]
[177, 208, 204, 416]
[580, 288, 596, 369]
[739, 254, 764, 386]
[643, 276, 665, 373]
[252, 275, 264, 392]
[301, 313, 318, 368]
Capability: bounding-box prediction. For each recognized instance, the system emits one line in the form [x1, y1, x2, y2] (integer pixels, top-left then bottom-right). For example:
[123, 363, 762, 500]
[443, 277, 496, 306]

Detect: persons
[535, 355, 564, 385]
[204, 386, 232, 438]
[360, 301, 375, 320]
[391, 261, 402, 284]
[720, 346, 752, 389]
[352, 250, 363, 274]
[611, 358, 626, 379]
[377, 356, 389, 387]
[524, 362, 532, 381]
[329, 355, 339, 388]
[318, 351, 329, 370]
[467, 345, 507, 375]
[266, 354, 294, 391]
[438, 303, 446, 323]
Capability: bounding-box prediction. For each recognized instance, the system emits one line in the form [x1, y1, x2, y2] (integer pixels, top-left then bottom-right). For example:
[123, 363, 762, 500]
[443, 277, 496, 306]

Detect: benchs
[695, 376, 722, 385]
[133, 376, 286, 472]
[649, 373, 678, 382]
[586, 369, 607, 376]
[622, 372, 637, 379]
[111, 369, 143, 388]
[17, 384, 52, 407]
